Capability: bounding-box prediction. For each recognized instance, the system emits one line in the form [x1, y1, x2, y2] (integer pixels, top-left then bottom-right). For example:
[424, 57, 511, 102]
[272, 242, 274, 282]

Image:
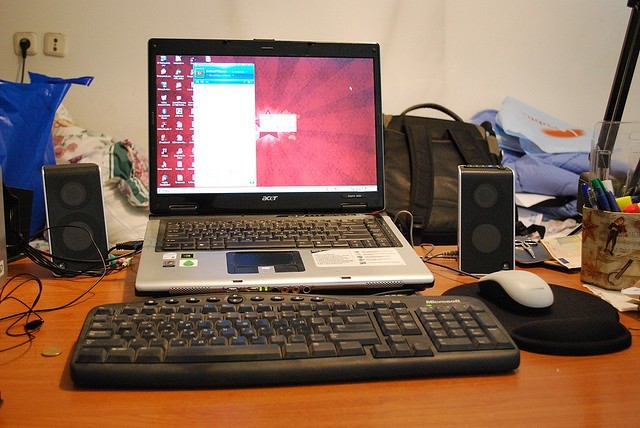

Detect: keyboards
[69, 292, 520, 389]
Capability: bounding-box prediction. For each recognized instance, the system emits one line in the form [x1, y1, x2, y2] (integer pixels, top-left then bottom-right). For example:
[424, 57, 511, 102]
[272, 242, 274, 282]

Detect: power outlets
[11, 30, 38, 56]
[42, 31, 67, 58]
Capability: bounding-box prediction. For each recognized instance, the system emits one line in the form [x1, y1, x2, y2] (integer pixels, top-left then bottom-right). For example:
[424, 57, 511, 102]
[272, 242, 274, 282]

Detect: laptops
[134, 36, 436, 293]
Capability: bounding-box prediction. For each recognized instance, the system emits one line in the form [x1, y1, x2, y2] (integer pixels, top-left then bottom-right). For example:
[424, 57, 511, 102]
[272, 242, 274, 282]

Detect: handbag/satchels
[0, 71, 94, 238]
[496, 95, 621, 156]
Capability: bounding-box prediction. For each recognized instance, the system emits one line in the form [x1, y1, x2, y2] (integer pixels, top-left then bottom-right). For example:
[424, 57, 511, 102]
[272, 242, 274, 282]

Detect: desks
[0, 243, 636, 426]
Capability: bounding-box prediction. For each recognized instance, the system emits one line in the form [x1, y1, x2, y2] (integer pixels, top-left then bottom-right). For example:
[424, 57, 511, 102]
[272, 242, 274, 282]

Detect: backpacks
[383, 103, 545, 246]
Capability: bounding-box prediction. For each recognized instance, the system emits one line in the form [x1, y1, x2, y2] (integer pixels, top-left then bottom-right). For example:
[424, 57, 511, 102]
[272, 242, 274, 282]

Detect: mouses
[478, 267, 555, 310]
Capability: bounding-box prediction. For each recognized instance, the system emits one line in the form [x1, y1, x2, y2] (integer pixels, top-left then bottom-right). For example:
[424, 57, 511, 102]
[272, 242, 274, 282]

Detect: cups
[577, 204, 640, 294]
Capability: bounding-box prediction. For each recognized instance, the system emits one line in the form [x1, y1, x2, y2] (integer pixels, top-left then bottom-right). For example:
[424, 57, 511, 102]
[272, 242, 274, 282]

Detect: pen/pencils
[591, 178, 610, 211]
[586, 188, 598, 209]
[606, 190, 621, 212]
[580, 183, 590, 209]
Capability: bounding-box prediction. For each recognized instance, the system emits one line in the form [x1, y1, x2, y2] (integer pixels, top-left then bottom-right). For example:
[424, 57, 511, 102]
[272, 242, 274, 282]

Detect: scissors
[513, 238, 538, 259]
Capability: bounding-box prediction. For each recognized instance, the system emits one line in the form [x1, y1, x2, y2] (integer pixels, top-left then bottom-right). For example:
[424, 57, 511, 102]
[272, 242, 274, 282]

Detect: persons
[604, 216, 628, 257]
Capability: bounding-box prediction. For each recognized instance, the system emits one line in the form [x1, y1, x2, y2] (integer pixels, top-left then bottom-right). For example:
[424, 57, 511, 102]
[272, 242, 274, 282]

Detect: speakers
[40, 162, 111, 277]
[456, 162, 516, 275]
